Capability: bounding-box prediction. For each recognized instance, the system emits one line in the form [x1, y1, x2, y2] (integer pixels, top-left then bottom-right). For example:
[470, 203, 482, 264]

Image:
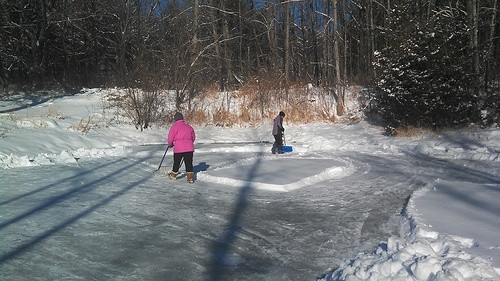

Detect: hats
[174, 111, 183, 120]
[279, 111, 285, 115]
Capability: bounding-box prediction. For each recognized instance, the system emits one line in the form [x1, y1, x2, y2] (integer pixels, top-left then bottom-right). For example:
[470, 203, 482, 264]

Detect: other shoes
[271, 148, 275, 154]
[278, 151, 284, 154]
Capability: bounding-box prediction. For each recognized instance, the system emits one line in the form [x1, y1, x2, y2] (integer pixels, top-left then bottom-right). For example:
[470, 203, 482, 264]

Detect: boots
[168, 170, 179, 180]
[186, 171, 194, 183]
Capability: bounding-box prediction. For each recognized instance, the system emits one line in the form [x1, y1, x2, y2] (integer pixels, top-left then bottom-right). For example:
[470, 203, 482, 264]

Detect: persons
[271, 111, 285, 154]
[168, 111, 196, 184]
